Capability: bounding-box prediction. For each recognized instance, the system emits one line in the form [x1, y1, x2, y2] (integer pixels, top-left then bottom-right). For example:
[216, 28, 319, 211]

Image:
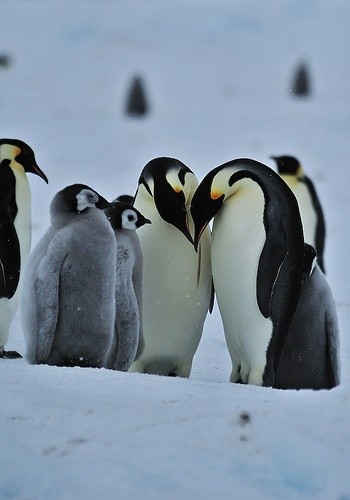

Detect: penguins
[113, 156, 215, 379]
[275, 242, 342, 393]
[102, 201, 152, 374]
[189, 156, 306, 392]
[270, 150, 327, 272]
[20, 183, 117, 369]
[1, 138, 49, 360]
